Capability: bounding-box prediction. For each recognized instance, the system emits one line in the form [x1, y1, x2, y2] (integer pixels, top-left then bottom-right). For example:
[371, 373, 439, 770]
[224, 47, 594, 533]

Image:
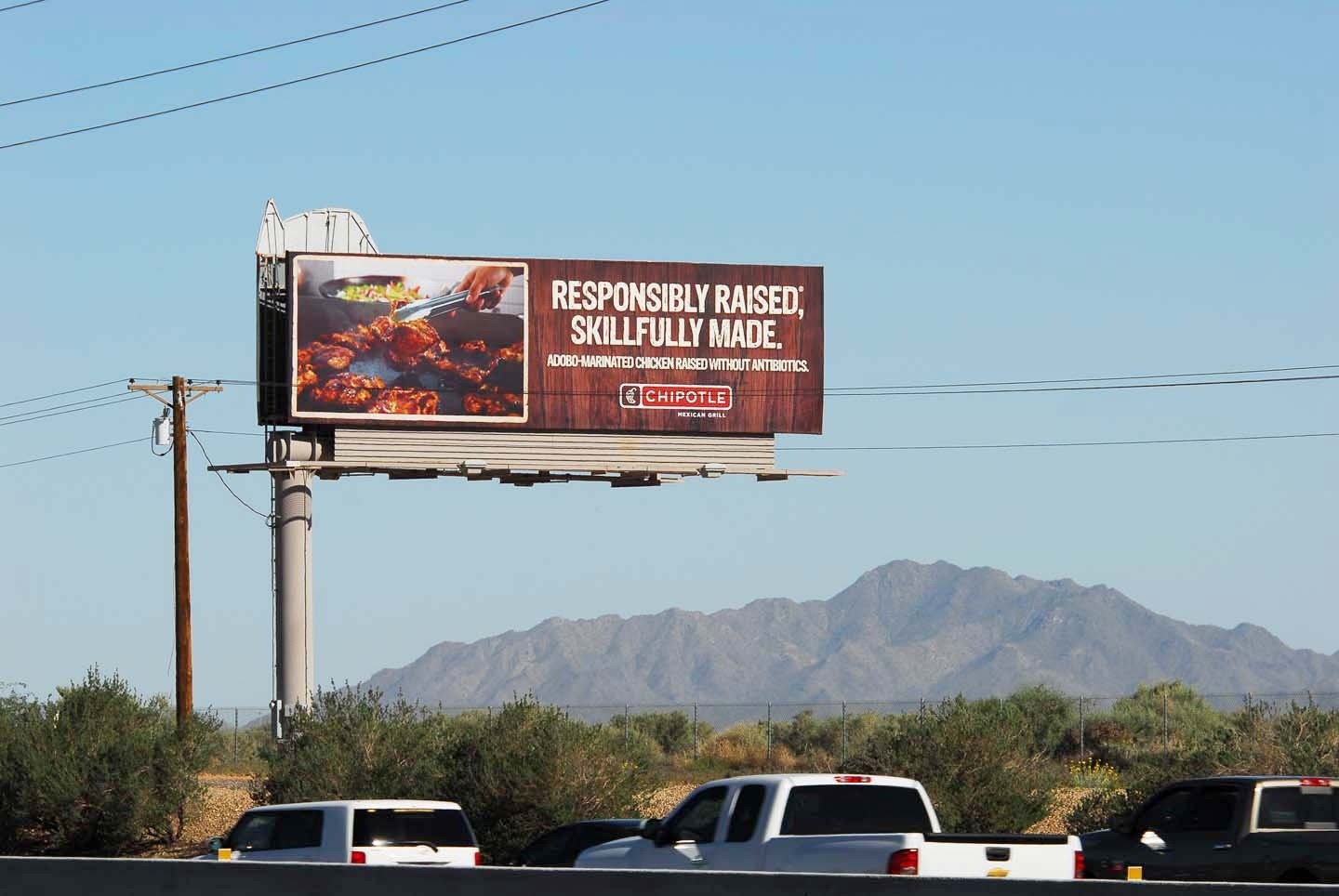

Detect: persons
[449, 267, 524, 317]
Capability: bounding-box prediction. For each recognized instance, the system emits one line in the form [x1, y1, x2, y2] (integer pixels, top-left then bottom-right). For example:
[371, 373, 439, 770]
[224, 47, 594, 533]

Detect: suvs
[190, 799, 482, 867]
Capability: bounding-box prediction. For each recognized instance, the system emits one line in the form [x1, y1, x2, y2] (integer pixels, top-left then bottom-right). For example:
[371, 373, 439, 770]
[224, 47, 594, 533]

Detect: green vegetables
[341, 282, 421, 302]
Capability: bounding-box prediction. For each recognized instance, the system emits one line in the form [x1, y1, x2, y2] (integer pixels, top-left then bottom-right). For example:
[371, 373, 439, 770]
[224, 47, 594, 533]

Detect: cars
[503, 817, 651, 867]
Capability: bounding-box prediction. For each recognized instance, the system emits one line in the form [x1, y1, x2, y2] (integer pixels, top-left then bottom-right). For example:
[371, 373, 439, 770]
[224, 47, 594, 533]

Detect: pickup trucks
[572, 772, 1087, 881]
[1077, 773, 1339, 885]
